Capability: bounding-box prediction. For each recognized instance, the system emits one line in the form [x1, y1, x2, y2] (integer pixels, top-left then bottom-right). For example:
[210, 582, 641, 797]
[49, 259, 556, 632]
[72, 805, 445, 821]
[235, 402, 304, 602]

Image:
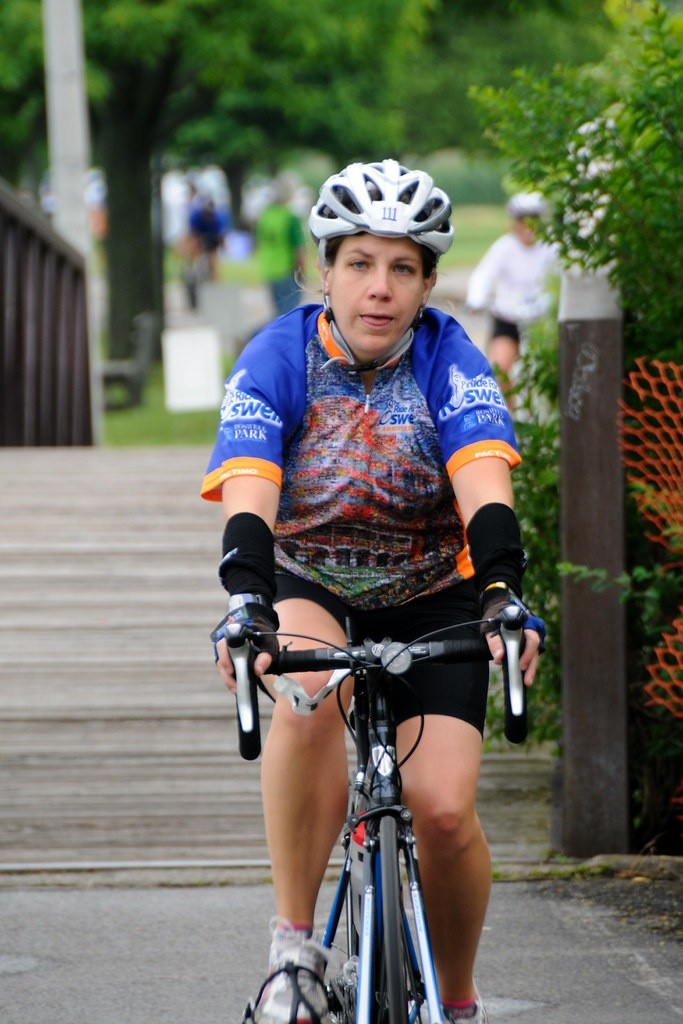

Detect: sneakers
[266, 928, 328, 1021]
[437, 999, 488, 1024]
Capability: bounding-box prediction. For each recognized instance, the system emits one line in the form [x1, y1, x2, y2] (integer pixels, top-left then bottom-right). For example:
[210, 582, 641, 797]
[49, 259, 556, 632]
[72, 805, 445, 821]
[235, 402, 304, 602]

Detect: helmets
[507, 191, 549, 218]
[309, 158, 454, 255]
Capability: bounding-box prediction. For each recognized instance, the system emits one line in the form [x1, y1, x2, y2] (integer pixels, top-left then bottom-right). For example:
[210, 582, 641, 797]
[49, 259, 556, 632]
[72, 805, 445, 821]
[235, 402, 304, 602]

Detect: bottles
[348, 810, 368, 896]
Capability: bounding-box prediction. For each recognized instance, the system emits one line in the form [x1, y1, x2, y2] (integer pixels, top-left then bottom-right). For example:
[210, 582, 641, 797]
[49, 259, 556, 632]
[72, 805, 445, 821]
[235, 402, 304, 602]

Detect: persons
[465, 186, 568, 418]
[17, 170, 311, 321]
[198, 158, 546, 1024]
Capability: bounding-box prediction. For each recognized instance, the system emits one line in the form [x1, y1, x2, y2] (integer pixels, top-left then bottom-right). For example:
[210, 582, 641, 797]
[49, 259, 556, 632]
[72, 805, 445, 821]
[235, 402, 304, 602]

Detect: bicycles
[224, 606, 528, 1023]
[470, 296, 556, 425]
